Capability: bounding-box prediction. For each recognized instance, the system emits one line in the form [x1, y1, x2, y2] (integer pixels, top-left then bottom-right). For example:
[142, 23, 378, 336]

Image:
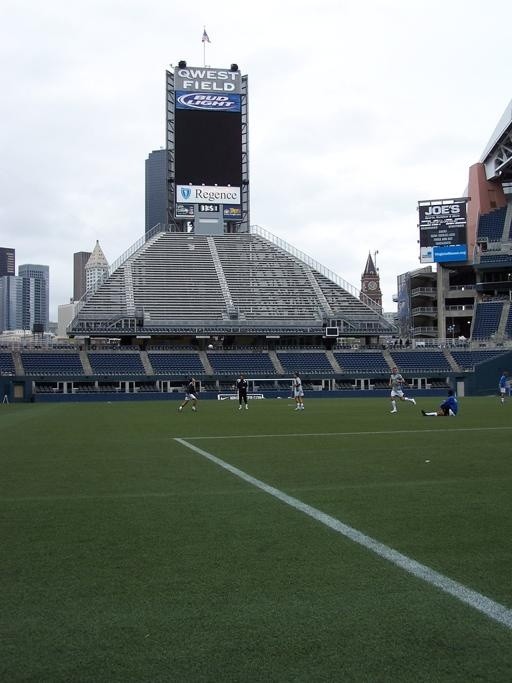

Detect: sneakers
[179, 406, 182, 411]
[391, 410, 397, 413]
[296, 406, 305, 410]
[422, 410, 425, 415]
[239, 407, 248, 410]
[192, 406, 195, 411]
[412, 398, 416, 405]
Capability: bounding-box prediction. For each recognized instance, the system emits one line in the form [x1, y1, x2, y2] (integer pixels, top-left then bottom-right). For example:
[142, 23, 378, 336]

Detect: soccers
[208, 345, 213, 349]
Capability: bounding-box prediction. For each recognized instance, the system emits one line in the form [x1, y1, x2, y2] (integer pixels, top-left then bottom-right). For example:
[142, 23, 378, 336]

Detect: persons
[499, 372, 507, 405]
[178, 375, 200, 415]
[292, 371, 304, 411]
[235, 375, 249, 410]
[420, 389, 458, 417]
[389, 365, 416, 414]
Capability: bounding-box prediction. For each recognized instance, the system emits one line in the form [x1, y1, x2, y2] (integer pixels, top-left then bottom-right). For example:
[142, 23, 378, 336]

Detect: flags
[201, 33, 212, 43]
